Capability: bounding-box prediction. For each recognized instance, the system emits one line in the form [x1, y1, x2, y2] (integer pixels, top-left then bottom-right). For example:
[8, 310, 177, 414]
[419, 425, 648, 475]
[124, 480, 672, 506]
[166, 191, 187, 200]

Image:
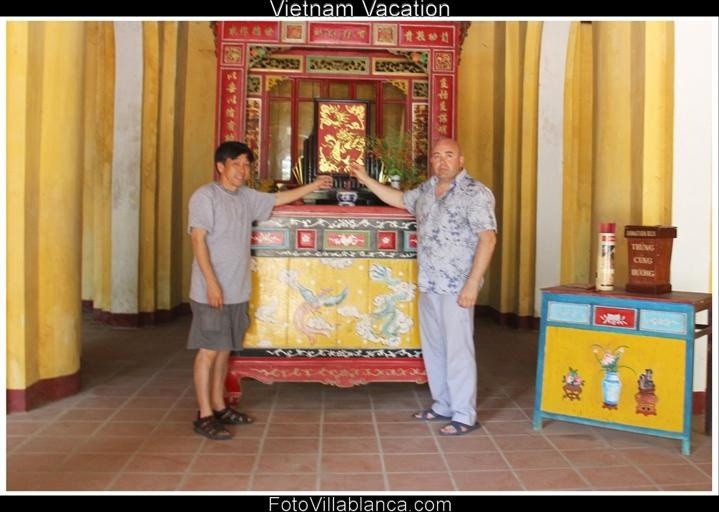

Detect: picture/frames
[311, 97, 372, 190]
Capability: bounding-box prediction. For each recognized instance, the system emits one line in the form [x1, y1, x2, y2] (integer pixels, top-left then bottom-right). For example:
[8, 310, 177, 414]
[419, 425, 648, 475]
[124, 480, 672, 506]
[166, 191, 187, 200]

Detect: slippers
[412, 408, 452, 421]
[439, 419, 481, 436]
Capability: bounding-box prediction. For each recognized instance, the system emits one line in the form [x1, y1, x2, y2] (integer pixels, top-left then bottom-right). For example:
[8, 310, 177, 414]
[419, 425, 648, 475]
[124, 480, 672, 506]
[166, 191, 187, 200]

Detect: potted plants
[359, 129, 425, 191]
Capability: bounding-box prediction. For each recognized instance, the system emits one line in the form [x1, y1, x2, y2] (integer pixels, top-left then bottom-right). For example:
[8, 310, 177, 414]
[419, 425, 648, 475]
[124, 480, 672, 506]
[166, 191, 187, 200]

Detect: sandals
[213, 406, 255, 425]
[192, 410, 232, 440]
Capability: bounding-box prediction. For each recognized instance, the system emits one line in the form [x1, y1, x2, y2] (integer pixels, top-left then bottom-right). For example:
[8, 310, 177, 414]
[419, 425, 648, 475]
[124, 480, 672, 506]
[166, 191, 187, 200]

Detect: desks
[536, 284, 711, 456]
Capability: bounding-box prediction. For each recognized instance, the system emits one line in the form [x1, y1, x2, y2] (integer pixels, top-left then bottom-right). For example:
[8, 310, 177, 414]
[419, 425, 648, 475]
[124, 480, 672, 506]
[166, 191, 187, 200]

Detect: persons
[184, 140, 336, 438]
[347, 137, 499, 436]
